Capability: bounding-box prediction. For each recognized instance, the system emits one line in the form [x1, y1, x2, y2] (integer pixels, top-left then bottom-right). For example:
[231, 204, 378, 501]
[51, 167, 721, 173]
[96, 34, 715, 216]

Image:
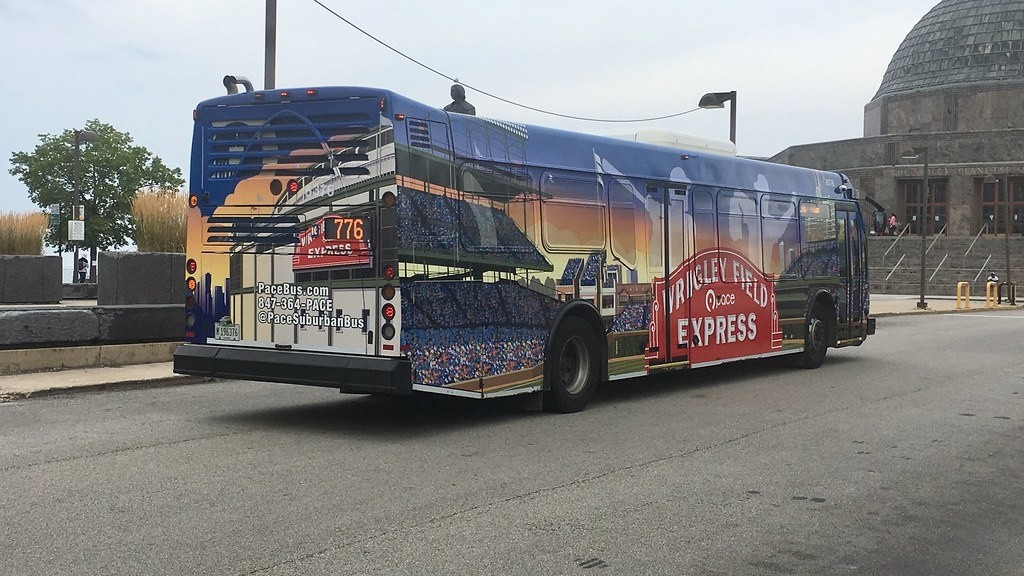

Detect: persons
[888, 213, 896, 236]
[987, 272, 999, 288]
[79, 254, 89, 283]
[444, 85, 475, 116]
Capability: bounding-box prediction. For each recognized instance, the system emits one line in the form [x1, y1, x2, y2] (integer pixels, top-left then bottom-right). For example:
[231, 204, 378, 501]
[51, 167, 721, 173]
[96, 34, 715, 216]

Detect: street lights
[70, 129, 101, 282]
[697, 91, 744, 144]
[984, 173, 1012, 303]
[901, 146, 928, 310]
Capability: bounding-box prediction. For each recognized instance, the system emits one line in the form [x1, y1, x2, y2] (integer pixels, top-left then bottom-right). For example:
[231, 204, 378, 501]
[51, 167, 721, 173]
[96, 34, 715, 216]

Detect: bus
[171, 86, 887, 413]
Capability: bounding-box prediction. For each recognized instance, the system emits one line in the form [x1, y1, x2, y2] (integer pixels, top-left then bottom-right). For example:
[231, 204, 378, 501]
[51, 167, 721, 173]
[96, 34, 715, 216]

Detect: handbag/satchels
[892, 222, 897, 227]
